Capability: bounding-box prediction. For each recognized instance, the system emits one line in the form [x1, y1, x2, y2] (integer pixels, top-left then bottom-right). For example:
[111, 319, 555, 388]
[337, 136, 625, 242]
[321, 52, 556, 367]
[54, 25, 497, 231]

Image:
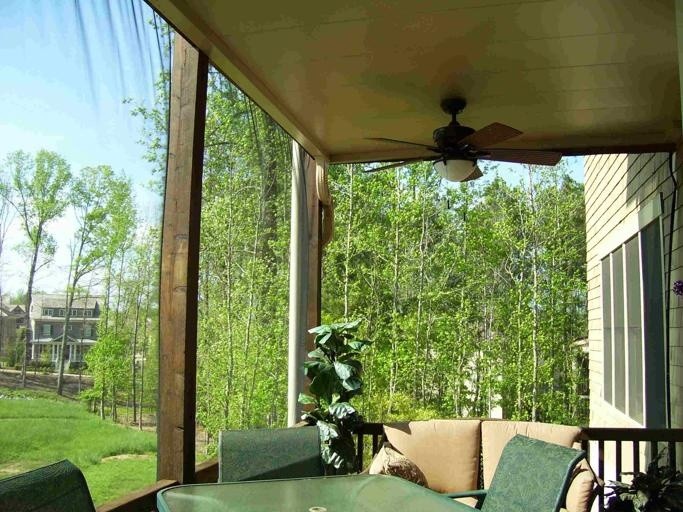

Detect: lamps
[432, 152, 477, 183]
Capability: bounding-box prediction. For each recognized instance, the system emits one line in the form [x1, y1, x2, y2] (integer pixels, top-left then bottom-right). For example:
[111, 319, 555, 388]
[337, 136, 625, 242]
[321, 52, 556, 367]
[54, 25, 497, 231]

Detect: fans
[359, 97, 562, 183]
